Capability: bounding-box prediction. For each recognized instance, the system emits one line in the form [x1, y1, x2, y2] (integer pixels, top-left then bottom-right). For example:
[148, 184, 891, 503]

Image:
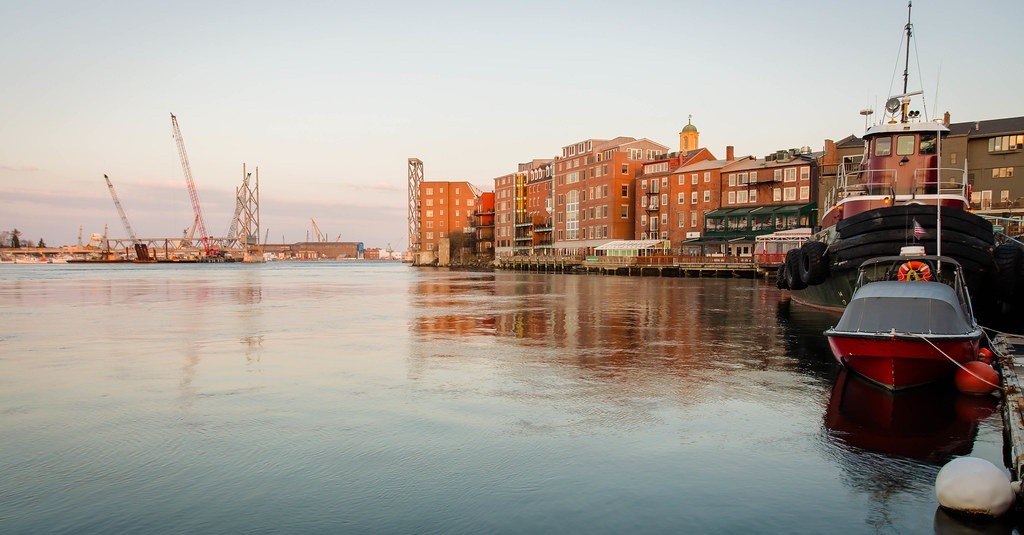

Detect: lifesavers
[784, 248, 808, 291]
[897, 260, 931, 282]
[799, 240, 830, 285]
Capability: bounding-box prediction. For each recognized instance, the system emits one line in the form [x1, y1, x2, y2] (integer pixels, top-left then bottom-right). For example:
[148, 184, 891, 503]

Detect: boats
[823, 246, 983, 393]
[778, 2, 1024, 347]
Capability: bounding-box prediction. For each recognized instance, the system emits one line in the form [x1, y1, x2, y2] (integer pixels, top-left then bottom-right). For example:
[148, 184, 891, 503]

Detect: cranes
[103, 172, 155, 263]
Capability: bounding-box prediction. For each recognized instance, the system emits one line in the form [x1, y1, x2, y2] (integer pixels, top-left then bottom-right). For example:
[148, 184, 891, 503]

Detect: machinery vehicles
[169, 111, 227, 267]
